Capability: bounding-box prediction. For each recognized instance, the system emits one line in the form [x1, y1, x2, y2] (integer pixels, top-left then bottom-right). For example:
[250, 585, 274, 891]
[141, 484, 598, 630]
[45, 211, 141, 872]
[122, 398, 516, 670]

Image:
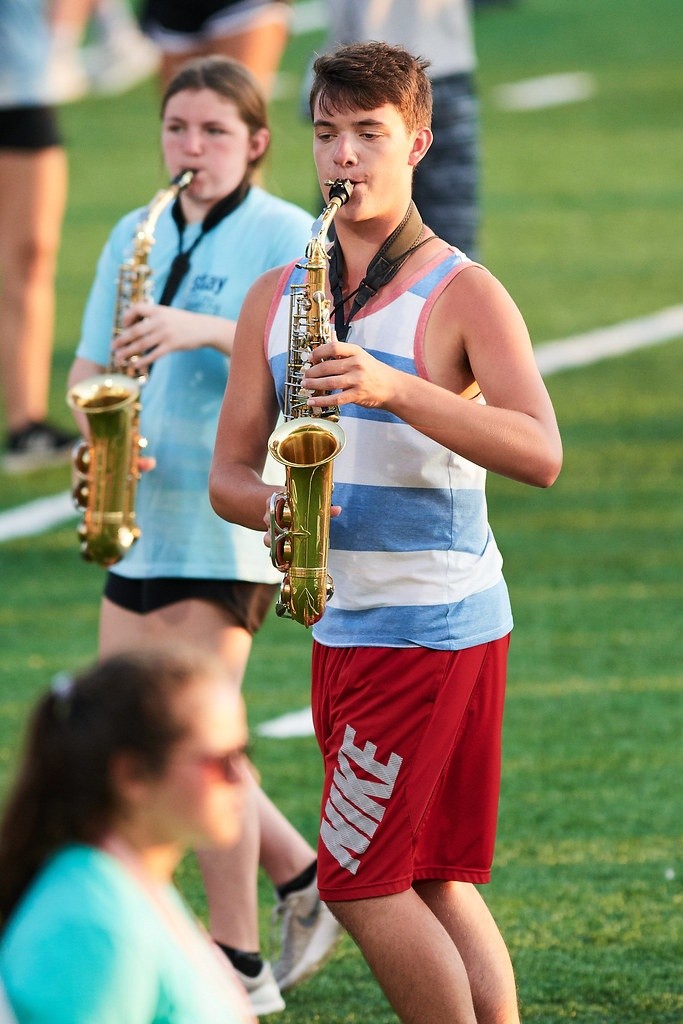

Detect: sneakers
[4, 421, 83, 475]
[237, 958, 285, 1018]
[274, 872, 343, 988]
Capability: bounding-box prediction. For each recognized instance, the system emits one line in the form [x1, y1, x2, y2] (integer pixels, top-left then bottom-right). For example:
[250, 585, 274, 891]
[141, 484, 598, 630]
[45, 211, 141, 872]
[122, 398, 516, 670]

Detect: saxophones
[266, 175, 356, 624]
[67, 167, 190, 571]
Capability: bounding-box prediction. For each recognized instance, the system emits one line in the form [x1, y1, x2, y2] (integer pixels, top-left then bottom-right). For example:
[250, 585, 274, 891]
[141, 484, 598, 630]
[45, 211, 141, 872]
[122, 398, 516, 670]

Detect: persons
[68, 47, 342, 1019]
[0, 0, 86, 479]
[212, 40, 568, 1024]
[4, 645, 253, 1022]
[328, 0, 483, 267]
[137, 0, 298, 188]
